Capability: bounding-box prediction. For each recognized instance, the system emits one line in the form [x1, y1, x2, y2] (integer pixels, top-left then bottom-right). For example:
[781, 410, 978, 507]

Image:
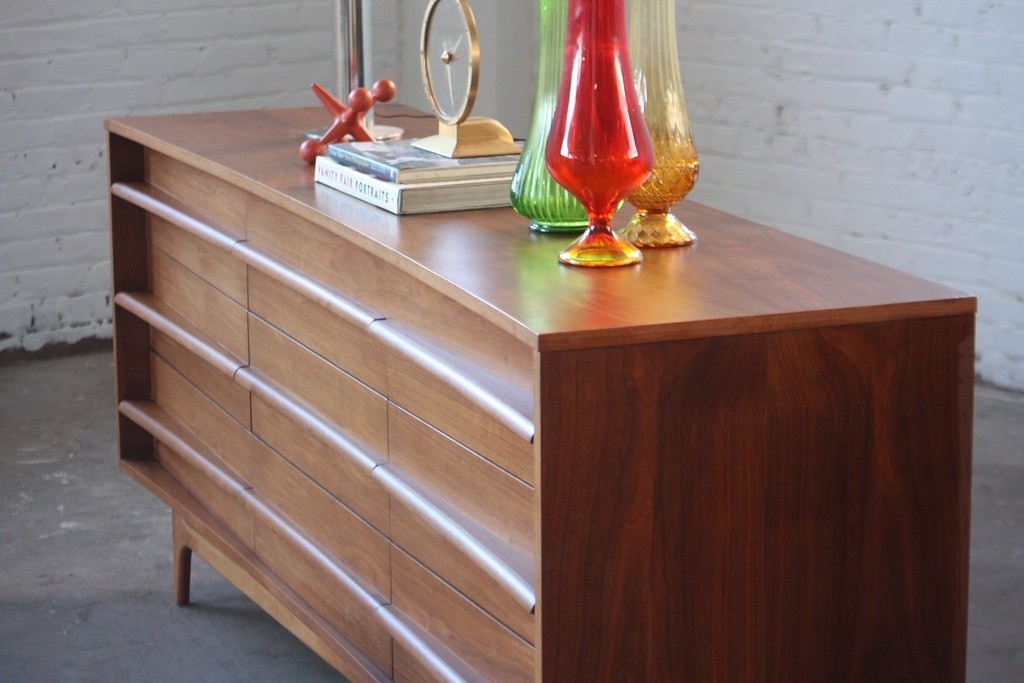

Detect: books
[315, 138, 522, 216]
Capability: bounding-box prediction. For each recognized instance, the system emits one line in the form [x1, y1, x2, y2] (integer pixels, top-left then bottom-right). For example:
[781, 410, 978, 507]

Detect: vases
[509, 0, 703, 268]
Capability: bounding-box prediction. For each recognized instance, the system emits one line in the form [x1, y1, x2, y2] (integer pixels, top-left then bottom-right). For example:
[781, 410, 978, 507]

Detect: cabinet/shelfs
[107, 101, 979, 683]
